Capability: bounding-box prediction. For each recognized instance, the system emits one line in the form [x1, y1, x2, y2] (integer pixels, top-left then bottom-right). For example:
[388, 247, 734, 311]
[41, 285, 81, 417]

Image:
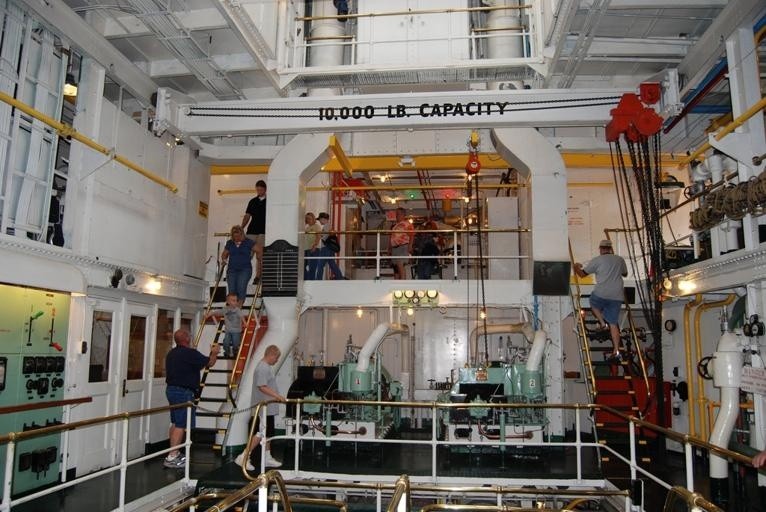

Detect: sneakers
[606, 353, 622, 363]
[594, 324, 610, 333]
[266, 456, 282, 468]
[164, 453, 186, 468]
[235, 455, 255, 470]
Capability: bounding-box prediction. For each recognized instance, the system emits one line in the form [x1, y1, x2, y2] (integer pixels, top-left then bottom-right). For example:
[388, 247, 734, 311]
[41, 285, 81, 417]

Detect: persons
[387, 208, 413, 279]
[220, 224, 264, 308]
[415, 226, 439, 279]
[574, 240, 629, 358]
[425, 222, 437, 238]
[162, 329, 221, 469]
[314, 212, 342, 279]
[304, 212, 320, 280]
[751, 447, 766, 468]
[233, 345, 286, 471]
[201, 293, 249, 357]
[239, 180, 265, 284]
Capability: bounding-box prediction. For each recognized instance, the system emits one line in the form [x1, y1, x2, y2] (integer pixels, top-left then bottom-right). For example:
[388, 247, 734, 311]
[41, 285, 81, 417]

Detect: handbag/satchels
[326, 235, 339, 252]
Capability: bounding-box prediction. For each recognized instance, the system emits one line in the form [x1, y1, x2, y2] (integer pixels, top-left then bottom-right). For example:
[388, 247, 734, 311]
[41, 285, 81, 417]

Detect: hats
[315, 213, 329, 221]
[600, 240, 611, 247]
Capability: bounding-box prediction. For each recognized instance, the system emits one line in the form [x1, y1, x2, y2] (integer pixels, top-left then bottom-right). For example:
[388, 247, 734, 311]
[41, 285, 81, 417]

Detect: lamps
[62, 70, 77, 97]
[391, 288, 440, 305]
